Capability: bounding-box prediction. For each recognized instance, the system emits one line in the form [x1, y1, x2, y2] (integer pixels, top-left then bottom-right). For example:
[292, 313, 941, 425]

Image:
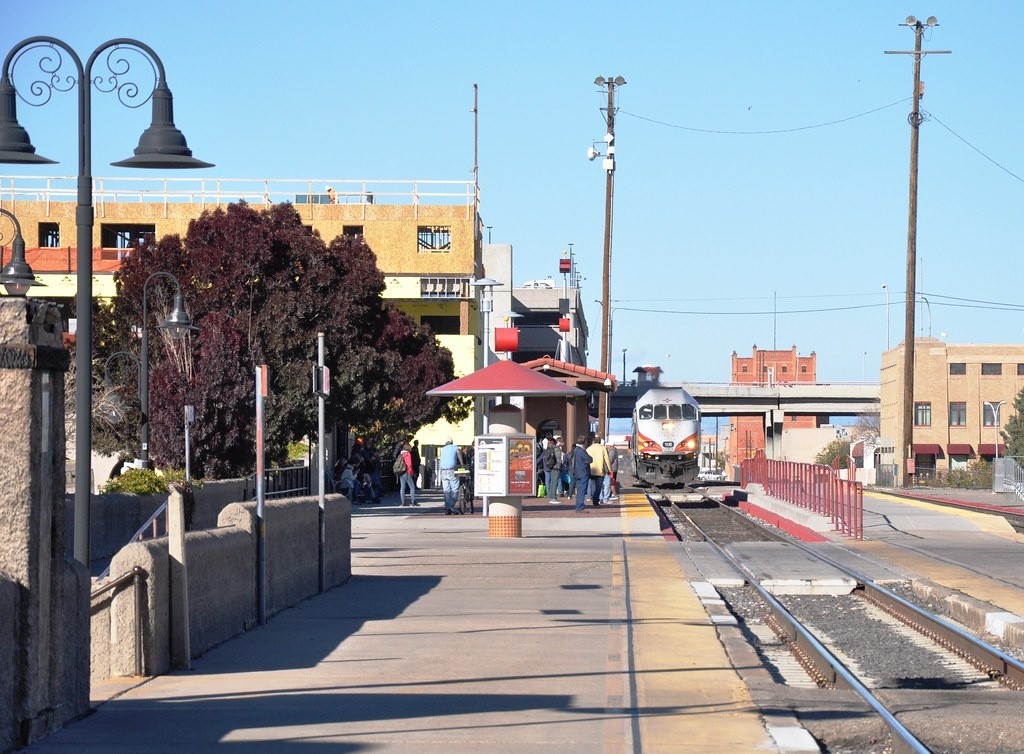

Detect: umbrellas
[426, 359, 586, 404]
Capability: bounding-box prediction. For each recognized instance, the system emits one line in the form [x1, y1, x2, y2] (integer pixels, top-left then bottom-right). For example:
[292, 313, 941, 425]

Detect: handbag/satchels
[537, 484, 545, 497]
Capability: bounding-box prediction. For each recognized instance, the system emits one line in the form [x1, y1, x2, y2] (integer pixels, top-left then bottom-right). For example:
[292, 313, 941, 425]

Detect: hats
[604, 440, 615, 446]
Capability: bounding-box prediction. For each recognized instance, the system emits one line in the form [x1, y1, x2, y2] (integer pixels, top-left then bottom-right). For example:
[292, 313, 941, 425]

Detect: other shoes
[448, 506, 458, 514]
[445, 510, 452, 515]
[549, 498, 562, 503]
[598, 499, 613, 504]
[402, 502, 410, 506]
[558, 494, 565, 497]
[576, 509, 589, 513]
[567, 497, 570, 499]
[609, 494, 619, 500]
[411, 501, 420, 506]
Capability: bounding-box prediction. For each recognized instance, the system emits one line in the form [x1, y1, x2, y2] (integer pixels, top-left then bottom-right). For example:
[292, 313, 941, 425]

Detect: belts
[442, 468, 454, 470]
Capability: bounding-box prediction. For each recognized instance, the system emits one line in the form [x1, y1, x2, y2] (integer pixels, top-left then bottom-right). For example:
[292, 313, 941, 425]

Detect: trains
[628, 387, 702, 488]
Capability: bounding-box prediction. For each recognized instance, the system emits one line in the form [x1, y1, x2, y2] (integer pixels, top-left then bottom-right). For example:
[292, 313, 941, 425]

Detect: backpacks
[393, 452, 408, 475]
[543, 447, 558, 469]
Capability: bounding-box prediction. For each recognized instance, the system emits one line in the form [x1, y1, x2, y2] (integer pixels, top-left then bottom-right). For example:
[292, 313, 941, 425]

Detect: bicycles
[456, 462, 476, 515]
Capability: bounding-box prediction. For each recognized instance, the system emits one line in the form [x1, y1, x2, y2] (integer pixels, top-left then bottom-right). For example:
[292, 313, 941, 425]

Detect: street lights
[983, 401, 1009, 459]
[882, 283, 890, 351]
[621, 347, 628, 384]
[103, 268, 204, 474]
[919, 295, 932, 336]
[0, 35, 220, 562]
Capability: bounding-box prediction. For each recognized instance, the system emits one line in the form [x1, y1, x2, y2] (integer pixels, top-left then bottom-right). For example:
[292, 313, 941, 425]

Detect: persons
[573, 435, 593, 513]
[440, 437, 475, 515]
[333, 443, 382, 505]
[536, 431, 620, 509]
[393, 436, 421, 506]
